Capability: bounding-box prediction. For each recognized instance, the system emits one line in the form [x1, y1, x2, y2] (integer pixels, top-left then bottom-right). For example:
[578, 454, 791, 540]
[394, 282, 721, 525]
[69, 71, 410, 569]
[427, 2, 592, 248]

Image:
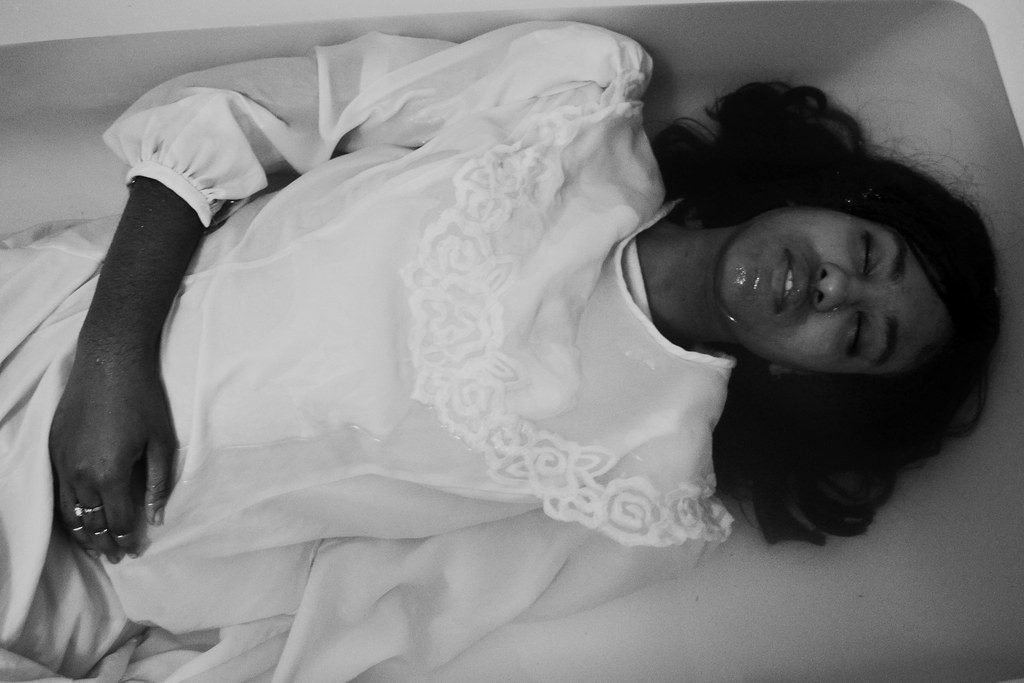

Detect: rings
[111, 531, 134, 539]
[71, 524, 84, 532]
[75, 502, 103, 517]
[88, 527, 109, 537]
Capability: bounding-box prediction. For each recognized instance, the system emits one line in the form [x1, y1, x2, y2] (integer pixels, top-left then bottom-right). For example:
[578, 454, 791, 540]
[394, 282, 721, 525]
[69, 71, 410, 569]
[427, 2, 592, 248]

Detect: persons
[2, 17, 999, 679]
